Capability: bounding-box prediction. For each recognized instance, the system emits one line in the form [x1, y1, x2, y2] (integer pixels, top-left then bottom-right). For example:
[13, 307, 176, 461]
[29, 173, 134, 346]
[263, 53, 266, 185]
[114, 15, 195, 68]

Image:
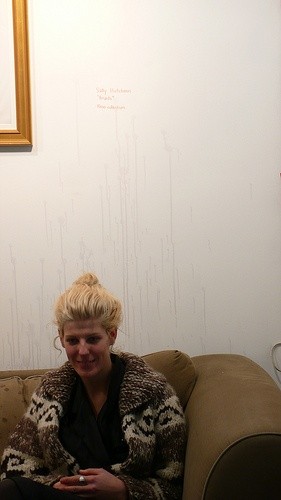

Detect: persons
[0, 273, 186, 500]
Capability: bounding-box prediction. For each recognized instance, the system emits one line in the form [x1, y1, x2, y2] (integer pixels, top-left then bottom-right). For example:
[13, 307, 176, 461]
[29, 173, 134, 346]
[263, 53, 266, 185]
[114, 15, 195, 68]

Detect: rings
[79, 475, 86, 486]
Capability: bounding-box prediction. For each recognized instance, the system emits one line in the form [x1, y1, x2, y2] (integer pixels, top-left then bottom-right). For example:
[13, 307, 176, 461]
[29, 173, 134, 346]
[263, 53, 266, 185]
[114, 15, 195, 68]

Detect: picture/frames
[0, 0, 33, 146]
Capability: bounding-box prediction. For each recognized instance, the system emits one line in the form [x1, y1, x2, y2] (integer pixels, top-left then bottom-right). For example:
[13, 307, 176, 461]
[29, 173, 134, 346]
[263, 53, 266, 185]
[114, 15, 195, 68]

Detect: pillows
[22, 350, 197, 409]
[0, 376, 29, 456]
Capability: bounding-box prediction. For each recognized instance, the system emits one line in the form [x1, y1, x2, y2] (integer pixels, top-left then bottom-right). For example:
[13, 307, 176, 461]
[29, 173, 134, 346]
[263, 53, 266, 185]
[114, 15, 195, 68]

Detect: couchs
[0, 354, 281, 500]
